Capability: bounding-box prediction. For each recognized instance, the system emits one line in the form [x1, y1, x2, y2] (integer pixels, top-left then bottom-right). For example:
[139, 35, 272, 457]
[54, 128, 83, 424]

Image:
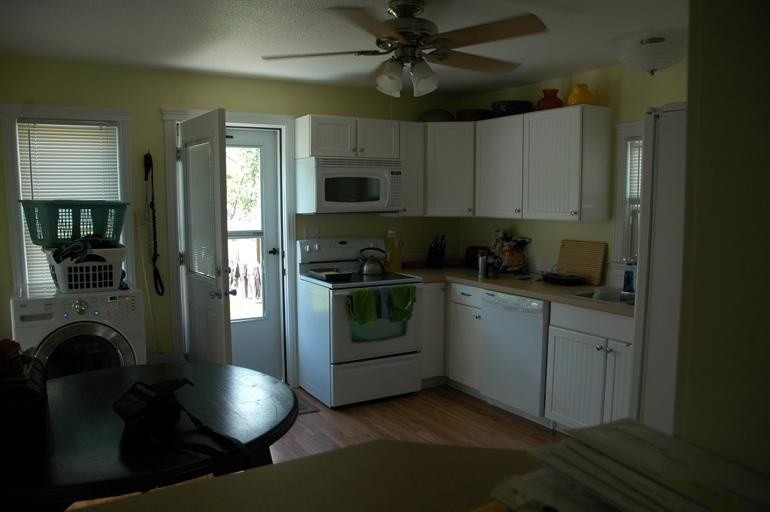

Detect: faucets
[623, 256, 635, 266]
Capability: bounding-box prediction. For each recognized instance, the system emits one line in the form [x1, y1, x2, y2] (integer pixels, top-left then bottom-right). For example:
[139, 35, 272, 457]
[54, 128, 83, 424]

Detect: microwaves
[297, 158, 405, 217]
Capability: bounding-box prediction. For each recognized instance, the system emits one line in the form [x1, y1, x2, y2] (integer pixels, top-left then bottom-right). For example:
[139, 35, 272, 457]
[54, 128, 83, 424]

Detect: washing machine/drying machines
[10, 290, 147, 381]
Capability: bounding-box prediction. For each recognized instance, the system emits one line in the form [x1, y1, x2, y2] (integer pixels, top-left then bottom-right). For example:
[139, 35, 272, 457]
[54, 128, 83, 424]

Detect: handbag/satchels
[112, 381, 180, 472]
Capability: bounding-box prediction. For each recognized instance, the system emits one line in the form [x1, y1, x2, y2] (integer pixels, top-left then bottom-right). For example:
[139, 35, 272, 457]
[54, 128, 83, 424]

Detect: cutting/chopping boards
[555, 238, 605, 287]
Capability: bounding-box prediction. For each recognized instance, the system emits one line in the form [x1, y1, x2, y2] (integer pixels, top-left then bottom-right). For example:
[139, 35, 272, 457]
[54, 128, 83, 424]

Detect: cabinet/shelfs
[473, 102, 619, 227]
[400, 120, 472, 218]
[420, 283, 484, 393]
[544, 301, 638, 436]
[293, 115, 400, 159]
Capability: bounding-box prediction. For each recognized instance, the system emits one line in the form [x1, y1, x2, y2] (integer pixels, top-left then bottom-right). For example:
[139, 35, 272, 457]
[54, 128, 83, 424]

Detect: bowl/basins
[541, 270, 592, 285]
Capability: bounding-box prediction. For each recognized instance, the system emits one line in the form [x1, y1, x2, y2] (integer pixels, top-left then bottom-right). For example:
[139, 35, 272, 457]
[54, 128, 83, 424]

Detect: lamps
[370, 41, 440, 100]
[611, 28, 690, 77]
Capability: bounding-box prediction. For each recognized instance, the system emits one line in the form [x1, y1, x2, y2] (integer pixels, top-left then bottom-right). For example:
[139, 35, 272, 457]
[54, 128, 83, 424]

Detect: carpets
[292, 389, 321, 416]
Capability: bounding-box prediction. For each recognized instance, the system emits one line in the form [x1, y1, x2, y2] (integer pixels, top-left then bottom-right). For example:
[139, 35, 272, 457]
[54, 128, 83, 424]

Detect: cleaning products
[496, 234, 532, 278]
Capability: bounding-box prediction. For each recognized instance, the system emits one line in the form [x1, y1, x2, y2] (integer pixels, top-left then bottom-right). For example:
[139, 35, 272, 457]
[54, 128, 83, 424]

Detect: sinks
[564, 286, 635, 306]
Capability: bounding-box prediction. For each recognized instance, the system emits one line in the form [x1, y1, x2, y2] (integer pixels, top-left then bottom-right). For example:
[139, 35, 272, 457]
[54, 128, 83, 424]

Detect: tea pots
[355, 246, 391, 278]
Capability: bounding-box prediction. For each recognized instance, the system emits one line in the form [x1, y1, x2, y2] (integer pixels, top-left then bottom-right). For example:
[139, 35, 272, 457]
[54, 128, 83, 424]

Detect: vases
[565, 84, 597, 107]
[537, 85, 564, 111]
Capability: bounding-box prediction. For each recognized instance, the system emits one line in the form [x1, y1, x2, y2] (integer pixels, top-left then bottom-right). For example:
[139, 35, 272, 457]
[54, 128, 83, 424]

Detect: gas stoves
[300, 266, 424, 289]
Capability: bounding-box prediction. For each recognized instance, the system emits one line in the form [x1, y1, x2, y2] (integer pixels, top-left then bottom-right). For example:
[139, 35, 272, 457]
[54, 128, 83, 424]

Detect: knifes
[432, 232, 448, 247]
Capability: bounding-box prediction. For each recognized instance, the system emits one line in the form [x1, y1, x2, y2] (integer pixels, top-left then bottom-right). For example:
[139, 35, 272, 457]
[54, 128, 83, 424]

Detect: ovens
[297, 283, 428, 411]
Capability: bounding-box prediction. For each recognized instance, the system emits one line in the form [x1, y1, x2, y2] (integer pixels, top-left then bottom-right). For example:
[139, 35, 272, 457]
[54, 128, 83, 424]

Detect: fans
[252, 0, 554, 102]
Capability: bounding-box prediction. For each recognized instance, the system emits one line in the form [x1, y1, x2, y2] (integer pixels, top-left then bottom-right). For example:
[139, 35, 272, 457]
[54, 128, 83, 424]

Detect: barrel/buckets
[385, 226, 411, 271]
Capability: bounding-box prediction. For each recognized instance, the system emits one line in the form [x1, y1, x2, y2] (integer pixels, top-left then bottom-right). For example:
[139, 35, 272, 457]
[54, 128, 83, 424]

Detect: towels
[344, 283, 418, 343]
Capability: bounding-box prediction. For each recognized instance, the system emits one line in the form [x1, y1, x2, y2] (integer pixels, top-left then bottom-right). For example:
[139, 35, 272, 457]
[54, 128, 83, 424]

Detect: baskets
[18, 201, 131, 293]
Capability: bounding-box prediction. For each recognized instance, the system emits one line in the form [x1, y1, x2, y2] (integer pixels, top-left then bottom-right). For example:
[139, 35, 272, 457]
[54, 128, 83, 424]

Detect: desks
[0, 354, 303, 500]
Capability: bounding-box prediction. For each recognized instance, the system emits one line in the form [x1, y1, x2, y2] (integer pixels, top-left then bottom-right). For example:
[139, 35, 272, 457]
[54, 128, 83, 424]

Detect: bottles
[476, 247, 504, 279]
[385, 228, 402, 270]
